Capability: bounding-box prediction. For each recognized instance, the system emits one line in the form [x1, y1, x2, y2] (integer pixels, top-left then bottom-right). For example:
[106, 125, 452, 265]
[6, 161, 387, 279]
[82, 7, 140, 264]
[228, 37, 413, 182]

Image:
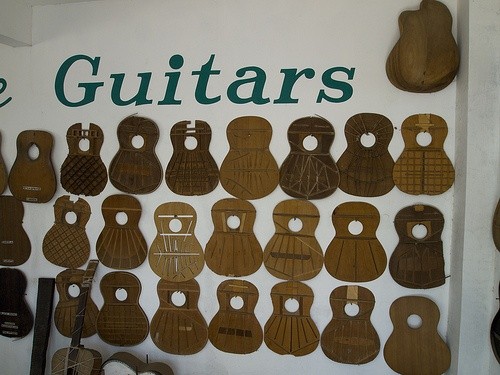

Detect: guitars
[51, 258, 101, 375]
